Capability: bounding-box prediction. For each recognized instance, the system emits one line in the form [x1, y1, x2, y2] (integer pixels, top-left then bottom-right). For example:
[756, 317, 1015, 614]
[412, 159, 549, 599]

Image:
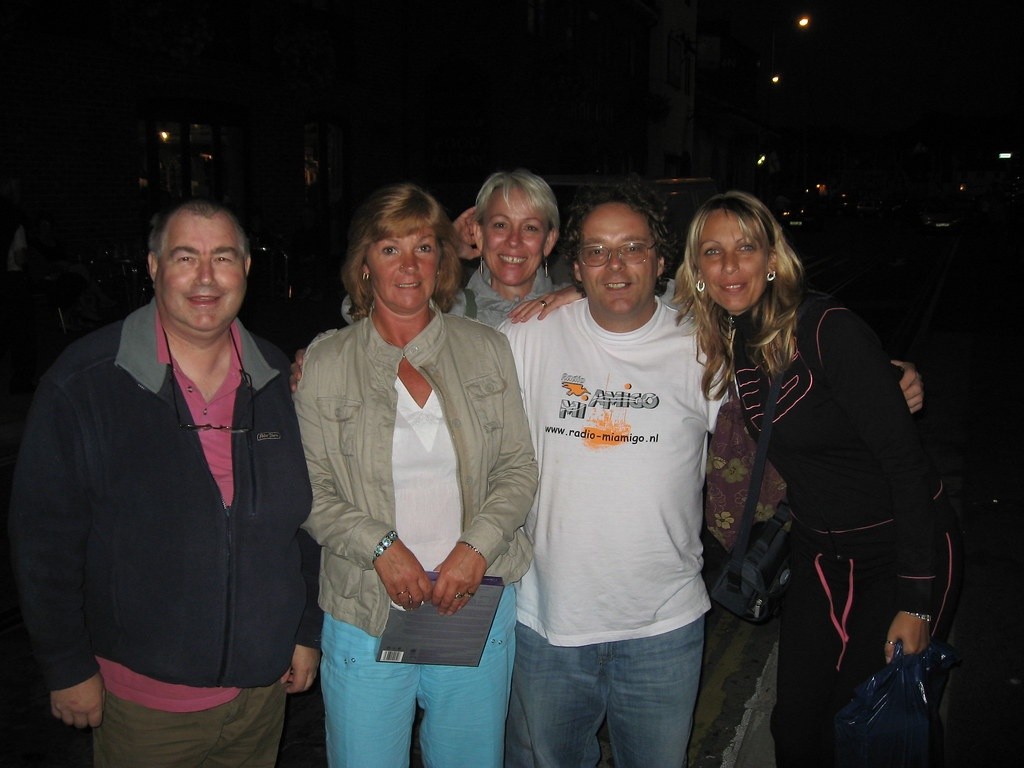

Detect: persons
[668, 191, 946, 768]
[492, 176, 925, 767]
[445, 169, 587, 333]
[291, 184, 541, 768]
[7, 195, 325, 768]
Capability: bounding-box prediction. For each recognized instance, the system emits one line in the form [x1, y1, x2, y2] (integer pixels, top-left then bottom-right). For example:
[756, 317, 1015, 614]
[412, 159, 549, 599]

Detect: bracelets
[460, 541, 484, 558]
[573, 282, 586, 298]
[371, 530, 398, 563]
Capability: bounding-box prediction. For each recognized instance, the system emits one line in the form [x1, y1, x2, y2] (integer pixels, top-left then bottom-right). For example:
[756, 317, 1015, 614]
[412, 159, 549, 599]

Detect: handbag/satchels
[710, 504, 794, 624]
[834, 634, 962, 768]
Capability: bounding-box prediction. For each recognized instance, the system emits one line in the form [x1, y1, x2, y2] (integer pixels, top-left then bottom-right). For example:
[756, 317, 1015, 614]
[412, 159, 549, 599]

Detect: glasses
[575, 242, 657, 267]
[165, 370, 255, 433]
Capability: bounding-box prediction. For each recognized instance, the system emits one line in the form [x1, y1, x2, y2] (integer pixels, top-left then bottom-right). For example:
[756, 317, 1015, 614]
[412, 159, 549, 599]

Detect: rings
[885, 640, 897, 646]
[540, 300, 548, 308]
[396, 587, 408, 596]
[454, 591, 475, 600]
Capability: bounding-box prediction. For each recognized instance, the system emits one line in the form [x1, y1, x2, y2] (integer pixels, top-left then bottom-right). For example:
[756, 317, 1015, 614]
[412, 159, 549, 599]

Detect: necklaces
[384, 339, 406, 358]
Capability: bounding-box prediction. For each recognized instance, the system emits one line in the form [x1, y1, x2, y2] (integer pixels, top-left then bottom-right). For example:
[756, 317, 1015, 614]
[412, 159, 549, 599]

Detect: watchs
[903, 610, 932, 623]
